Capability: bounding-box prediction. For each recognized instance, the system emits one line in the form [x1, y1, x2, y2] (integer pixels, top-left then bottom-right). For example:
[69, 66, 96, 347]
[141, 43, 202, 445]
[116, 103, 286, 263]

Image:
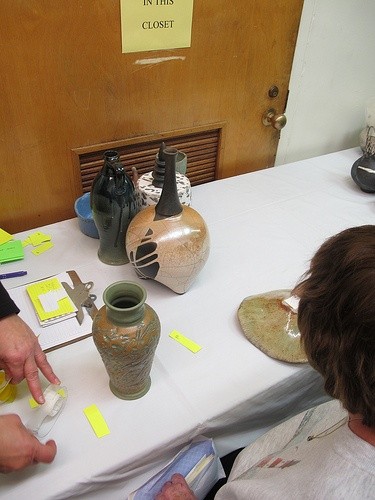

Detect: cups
[175, 151, 187, 176]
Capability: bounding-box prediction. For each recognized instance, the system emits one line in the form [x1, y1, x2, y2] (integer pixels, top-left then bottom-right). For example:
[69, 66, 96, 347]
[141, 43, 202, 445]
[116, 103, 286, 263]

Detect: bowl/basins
[74, 192, 99, 240]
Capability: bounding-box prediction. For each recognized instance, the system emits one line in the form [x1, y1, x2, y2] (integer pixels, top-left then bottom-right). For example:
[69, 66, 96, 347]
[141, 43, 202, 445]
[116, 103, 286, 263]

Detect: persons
[153, 225, 375, 500]
[0, 281, 60, 475]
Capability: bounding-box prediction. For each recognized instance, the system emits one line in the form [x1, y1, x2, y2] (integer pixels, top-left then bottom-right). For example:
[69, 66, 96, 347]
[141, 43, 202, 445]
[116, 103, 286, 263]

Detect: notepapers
[0, 240, 24, 264]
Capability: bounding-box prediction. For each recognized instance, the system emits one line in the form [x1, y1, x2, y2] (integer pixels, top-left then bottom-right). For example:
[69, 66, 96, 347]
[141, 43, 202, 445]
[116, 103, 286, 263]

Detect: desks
[0, 146, 375, 500]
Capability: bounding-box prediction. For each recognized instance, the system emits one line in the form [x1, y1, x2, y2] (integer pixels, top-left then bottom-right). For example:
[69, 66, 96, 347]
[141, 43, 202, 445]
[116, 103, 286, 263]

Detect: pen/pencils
[0, 271, 27, 279]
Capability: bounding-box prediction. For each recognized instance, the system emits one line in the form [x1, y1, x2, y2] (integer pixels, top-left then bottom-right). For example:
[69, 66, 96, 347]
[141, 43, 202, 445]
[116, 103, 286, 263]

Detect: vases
[126, 149, 210, 297]
[91, 280, 161, 401]
[90, 149, 138, 265]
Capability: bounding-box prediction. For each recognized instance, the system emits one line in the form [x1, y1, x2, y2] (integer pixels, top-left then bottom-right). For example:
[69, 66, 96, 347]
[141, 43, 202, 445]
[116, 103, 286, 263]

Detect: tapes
[39, 384, 68, 420]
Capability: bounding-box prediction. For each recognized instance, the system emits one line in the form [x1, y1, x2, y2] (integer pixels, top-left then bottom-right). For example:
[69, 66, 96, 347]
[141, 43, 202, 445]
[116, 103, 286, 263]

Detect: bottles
[90, 151, 140, 266]
[135, 141, 193, 211]
[91, 280, 161, 401]
[350, 151, 375, 194]
[125, 147, 211, 295]
